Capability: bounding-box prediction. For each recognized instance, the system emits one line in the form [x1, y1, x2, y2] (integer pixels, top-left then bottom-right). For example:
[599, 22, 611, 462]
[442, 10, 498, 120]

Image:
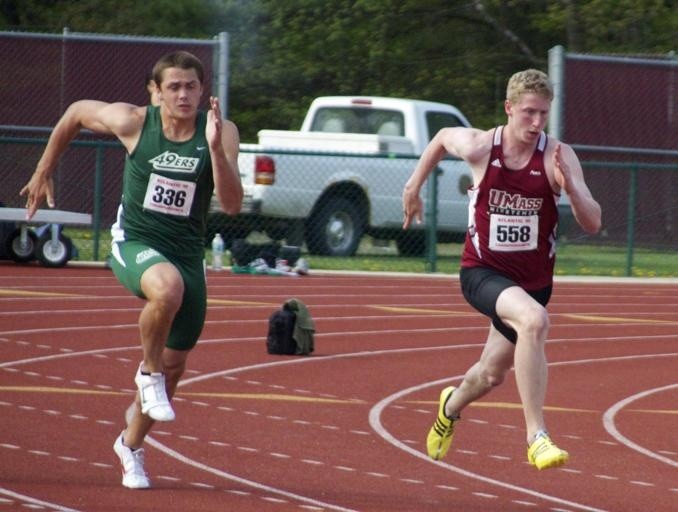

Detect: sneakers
[134, 361, 175, 422]
[249, 258, 269, 273]
[291, 258, 308, 275]
[274, 260, 291, 272]
[425, 386, 460, 462]
[112, 429, 149, 489]
[526, 433, 568, 471]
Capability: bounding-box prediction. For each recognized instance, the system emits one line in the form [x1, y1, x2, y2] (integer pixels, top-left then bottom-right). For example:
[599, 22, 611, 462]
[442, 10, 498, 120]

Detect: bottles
[212, 234, 224, 272]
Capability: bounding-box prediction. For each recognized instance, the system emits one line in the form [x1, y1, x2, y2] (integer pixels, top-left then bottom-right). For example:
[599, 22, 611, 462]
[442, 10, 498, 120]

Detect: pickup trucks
[212, 91, 578, 258]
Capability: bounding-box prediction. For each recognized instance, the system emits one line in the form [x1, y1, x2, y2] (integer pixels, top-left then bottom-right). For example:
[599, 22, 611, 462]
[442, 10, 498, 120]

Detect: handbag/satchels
[266, 305, 297, 354]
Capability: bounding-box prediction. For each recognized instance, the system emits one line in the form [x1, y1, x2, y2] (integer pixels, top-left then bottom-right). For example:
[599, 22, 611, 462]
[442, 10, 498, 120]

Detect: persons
[18, 51, 242, 490]
[401, 68, 601, 471]
